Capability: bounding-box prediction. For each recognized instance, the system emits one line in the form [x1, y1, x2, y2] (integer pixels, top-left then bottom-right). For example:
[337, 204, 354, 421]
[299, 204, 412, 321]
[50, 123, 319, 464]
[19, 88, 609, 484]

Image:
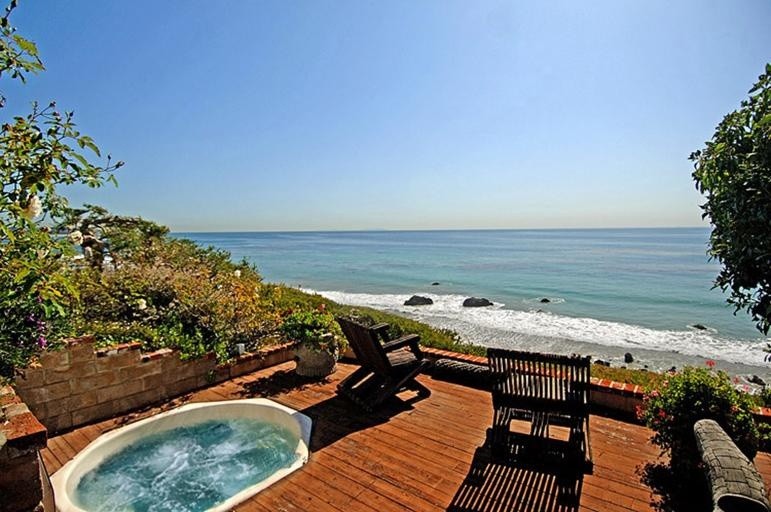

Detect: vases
[291, 344, 337, 375]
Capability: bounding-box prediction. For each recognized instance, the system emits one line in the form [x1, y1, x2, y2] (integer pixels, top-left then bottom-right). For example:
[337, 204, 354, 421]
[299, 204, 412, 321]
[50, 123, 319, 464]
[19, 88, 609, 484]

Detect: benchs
[485, 348, 591, 461]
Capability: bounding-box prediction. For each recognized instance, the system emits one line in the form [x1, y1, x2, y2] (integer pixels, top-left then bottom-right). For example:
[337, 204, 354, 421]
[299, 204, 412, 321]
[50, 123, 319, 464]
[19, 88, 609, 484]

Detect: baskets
[293, 342, 339, 377]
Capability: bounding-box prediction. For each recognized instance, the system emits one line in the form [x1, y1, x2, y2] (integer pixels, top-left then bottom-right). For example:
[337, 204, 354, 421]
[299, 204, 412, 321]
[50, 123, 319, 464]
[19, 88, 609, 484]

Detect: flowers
[278, 305, 350, 365]
[639, 359, 770, 457]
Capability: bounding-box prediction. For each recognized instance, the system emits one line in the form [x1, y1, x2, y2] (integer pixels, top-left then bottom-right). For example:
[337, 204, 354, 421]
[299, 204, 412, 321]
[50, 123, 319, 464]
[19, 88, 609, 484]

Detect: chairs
[333, 314, 430, 412]
